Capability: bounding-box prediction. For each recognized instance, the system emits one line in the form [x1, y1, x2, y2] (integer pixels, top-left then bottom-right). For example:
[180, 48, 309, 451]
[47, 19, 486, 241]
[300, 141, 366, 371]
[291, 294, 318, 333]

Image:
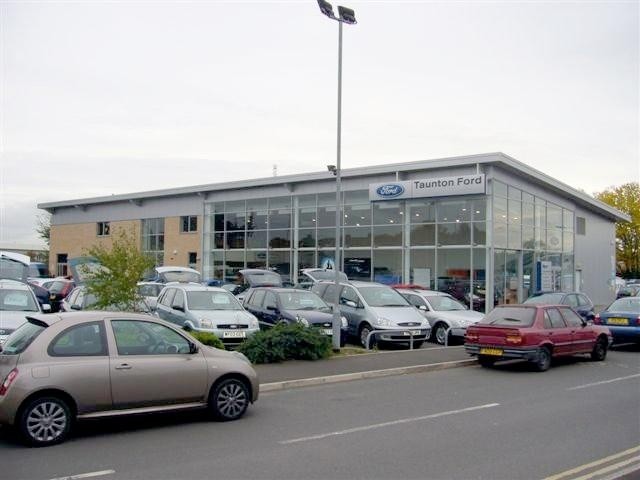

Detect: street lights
[317, 0, 358, 352]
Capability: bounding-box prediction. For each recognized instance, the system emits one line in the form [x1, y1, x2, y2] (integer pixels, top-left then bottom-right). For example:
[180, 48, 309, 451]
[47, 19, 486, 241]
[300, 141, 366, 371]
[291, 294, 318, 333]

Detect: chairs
[79, 327, 102, 353]
